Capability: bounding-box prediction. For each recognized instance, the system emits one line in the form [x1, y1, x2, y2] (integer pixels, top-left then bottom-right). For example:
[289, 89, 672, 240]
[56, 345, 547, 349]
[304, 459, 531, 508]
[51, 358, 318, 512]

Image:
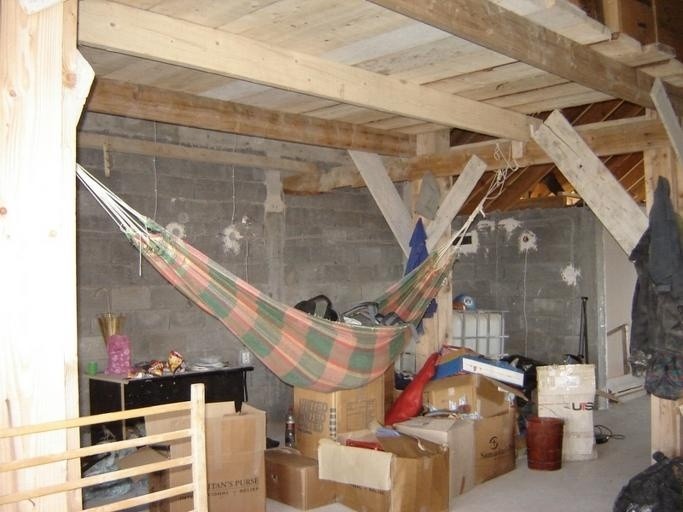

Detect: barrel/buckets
[526, 417, 565, 471]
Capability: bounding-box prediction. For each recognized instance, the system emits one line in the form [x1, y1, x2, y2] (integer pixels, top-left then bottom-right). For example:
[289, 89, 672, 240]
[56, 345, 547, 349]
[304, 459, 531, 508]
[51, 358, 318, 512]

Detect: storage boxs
[535, 362, 600, 464]
[421, 350, 529, 488]
[315, 426, 451, 512]
[116, 399, 268, 512]
[262, 447, 338, 511]
[280, 372, 386, 459]
[383, 357, 397, 420]
[392, 406, 476, 502]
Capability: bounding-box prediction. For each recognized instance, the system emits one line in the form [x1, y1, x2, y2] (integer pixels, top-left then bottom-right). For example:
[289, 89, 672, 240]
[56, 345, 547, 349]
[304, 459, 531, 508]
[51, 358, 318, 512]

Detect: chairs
[2, 381, 209, 512]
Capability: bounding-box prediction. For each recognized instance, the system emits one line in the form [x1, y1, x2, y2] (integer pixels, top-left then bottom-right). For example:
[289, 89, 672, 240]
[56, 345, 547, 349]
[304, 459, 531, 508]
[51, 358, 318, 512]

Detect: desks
[84, 362, 255, 441]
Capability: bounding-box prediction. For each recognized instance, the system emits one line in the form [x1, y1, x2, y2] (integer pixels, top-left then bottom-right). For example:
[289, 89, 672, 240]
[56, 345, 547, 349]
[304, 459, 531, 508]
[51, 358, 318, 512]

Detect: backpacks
[611, 453, 683, 508]
